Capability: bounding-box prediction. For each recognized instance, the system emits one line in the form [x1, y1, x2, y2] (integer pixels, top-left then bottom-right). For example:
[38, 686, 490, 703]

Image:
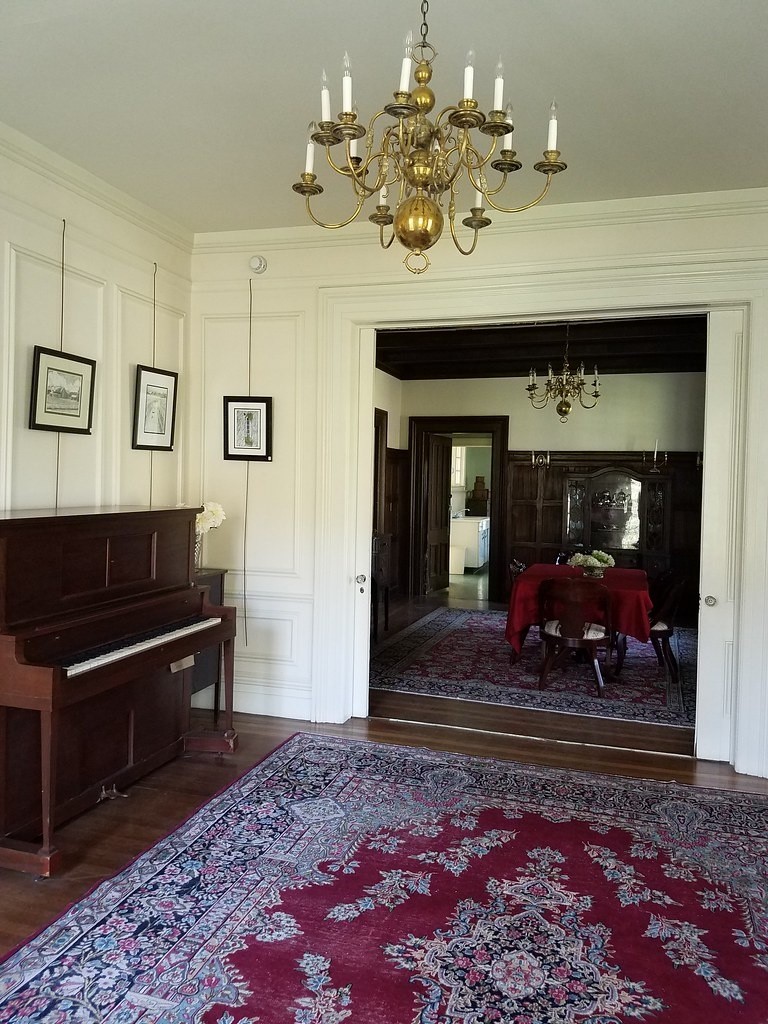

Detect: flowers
[180, 503, 226, 533]
[569, 550, 616, 567]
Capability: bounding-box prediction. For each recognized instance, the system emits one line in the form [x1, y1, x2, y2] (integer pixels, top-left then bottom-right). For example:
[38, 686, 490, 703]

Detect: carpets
[368, 606, 697, 731]
[1, 732, 768, 1024]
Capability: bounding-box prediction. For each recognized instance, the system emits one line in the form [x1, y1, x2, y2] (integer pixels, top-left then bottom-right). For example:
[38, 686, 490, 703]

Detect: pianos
[0, 502, 240, 880]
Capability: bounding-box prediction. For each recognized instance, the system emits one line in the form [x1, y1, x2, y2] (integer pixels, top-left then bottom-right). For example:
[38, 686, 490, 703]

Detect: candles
[654, 439, 659, 463]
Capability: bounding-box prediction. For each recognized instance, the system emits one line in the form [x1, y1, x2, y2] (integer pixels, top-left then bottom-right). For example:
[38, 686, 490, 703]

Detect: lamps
[291, 0, 567, 274]
[526, 326, 602, 423]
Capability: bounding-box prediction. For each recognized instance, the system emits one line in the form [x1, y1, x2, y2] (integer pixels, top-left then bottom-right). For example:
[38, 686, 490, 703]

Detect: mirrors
[565, 467, 648, 552]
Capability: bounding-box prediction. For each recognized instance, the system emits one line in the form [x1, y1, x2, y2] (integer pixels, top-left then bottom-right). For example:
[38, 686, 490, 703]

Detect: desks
[507, 563, 654, 676]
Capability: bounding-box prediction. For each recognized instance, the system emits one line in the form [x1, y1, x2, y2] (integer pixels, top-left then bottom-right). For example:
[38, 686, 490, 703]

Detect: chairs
[614, 567, 692, 676]
[557, 550, 592, 570]
[508, 559, 546, 651]
[538, 578, 613, 697]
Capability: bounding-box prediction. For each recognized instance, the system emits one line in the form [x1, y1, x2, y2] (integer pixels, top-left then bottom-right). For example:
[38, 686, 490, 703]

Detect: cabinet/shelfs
[448, 518, 489, 571]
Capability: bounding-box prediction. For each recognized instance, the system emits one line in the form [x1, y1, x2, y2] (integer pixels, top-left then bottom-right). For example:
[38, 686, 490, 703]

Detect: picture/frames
[29, 345, 96, 435]
[222, 395, 273, 461]
[131, 364, 178, 451]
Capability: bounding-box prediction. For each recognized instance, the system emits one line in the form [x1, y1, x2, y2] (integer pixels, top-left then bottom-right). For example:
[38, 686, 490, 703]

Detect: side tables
[189, 568, 229, 730]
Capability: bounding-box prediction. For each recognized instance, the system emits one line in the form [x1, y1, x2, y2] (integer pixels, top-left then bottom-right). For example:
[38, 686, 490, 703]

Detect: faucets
[456, 509, 470, 516]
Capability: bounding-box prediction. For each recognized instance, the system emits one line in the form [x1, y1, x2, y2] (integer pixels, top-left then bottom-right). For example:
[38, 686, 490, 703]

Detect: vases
[578, 565, 610, 580]
[194, 534, 201, 573]
